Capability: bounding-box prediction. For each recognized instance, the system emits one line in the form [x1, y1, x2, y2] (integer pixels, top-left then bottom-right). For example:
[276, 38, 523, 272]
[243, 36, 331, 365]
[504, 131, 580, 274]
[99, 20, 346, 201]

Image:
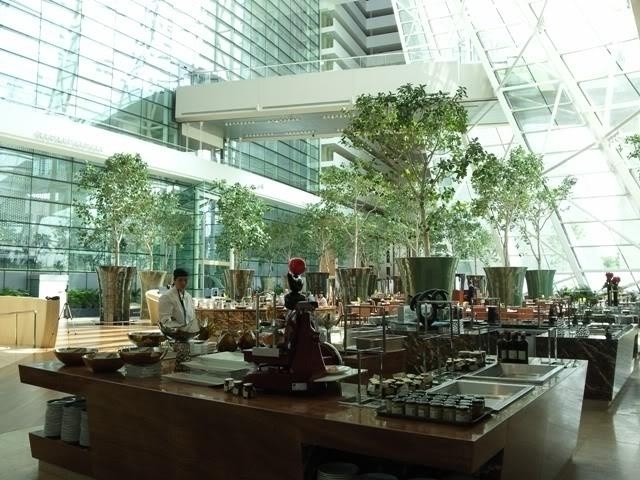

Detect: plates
[46, 396, 88, 447]
[315, 461, 396, 479]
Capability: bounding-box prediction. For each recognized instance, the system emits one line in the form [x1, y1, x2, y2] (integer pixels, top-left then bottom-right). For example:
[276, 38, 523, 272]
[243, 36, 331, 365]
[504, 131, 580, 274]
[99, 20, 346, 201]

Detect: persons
[159, 270, 199, 333]
[316, 291, 327, 307]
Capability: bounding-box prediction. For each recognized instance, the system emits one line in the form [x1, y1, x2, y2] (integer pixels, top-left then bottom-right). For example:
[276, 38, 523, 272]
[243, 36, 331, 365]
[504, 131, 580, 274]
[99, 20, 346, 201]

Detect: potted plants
[338, 83, 578, 306]
[210, 176, 272, 302]
[74, 154, 194, 326]
[282, 163, 406, 300]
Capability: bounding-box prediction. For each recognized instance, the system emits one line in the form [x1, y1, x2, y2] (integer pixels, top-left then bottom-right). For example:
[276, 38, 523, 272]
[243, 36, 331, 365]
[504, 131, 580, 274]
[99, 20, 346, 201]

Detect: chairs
[195, 293, 572, 326]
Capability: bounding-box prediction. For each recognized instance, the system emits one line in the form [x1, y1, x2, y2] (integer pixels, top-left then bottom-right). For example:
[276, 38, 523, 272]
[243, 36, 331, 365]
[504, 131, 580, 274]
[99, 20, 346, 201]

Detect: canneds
[386, 389, 485, 422]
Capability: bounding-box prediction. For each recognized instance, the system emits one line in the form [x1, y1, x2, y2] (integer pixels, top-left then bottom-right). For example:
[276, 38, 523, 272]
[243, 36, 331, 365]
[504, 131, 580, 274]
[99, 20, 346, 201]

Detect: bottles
[495, 332, 528, 362]
[223, 376, 254, 401]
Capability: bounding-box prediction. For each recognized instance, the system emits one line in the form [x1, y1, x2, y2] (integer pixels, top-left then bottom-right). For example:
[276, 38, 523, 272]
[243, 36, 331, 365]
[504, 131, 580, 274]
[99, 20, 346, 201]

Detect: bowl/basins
[53, 332, 168, 372]
